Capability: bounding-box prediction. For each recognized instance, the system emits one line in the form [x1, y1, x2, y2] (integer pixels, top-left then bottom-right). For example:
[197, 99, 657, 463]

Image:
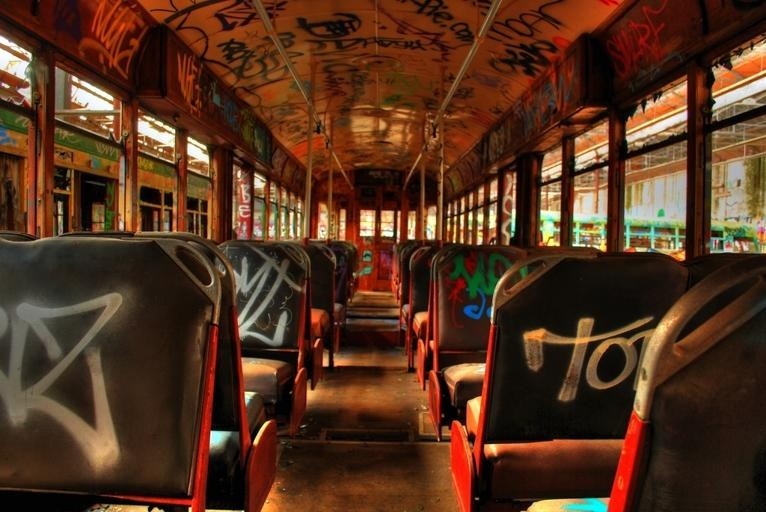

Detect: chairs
[389, 240, 766, 511]
[1, 230, 360, 512]
